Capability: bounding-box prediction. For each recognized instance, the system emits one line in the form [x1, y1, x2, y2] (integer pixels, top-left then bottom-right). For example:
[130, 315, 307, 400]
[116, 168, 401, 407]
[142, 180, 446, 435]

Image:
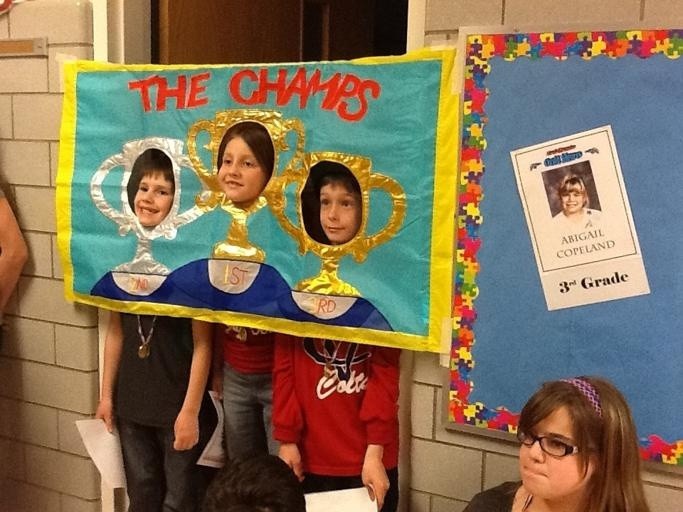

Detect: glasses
[516, 425, 582, 458]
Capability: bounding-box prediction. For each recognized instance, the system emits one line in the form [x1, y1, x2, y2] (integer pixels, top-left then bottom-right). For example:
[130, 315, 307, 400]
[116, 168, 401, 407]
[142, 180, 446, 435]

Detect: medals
[233, 326, 248, 343]
[318, 340, 345, 379]
[134, 313, 158, 359]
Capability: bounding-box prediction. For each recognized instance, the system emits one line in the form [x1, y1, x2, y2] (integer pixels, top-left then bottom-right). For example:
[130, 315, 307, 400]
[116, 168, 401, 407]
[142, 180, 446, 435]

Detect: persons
[95, 156, 212, 512]
[555, 174, 603, 228]
[465, 376, 649, 512]
[273, 170, 400, 511]
[0, 189, 28, 345]
[203, 450, 306, 511]
[209, 123, 280, 457]
